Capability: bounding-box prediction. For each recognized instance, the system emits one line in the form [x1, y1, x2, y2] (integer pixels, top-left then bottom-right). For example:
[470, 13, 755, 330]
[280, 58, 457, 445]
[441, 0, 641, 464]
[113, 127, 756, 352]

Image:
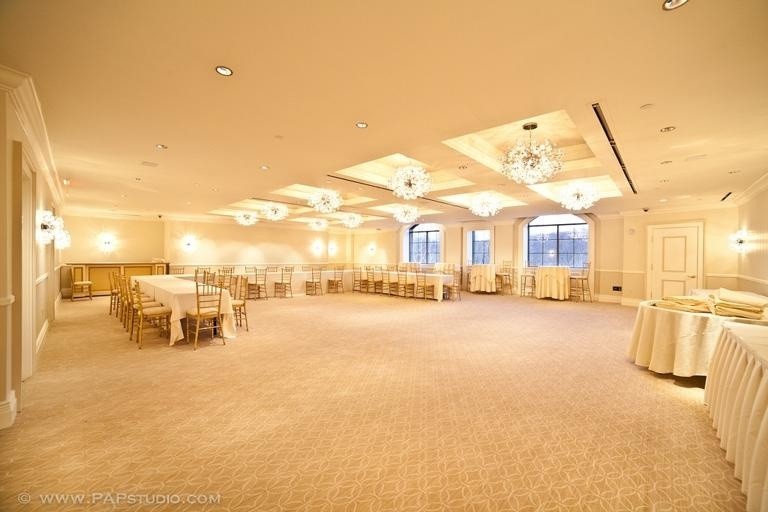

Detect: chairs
[70, 266, 93, 302]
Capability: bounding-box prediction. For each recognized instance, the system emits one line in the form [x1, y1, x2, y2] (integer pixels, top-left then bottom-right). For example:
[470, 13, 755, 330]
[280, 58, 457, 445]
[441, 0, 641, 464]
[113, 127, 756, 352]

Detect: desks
[703, 321, 768, 512]
[690, 288, 756, 296]
[627, 296, 768, 379]
